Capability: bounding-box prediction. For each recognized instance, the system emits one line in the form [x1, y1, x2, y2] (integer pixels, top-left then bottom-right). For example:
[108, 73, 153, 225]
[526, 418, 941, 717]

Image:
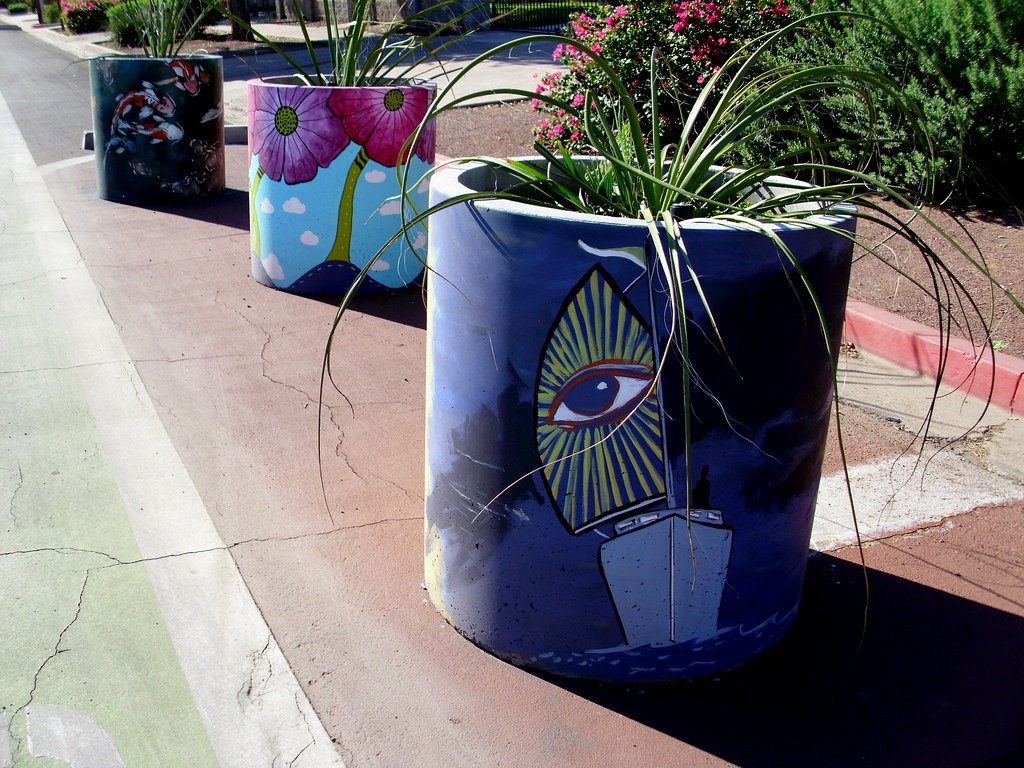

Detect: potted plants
[208, 0, 523, 297]
[319, 0, 1024, 681]
[88, 0, 226, 204]
